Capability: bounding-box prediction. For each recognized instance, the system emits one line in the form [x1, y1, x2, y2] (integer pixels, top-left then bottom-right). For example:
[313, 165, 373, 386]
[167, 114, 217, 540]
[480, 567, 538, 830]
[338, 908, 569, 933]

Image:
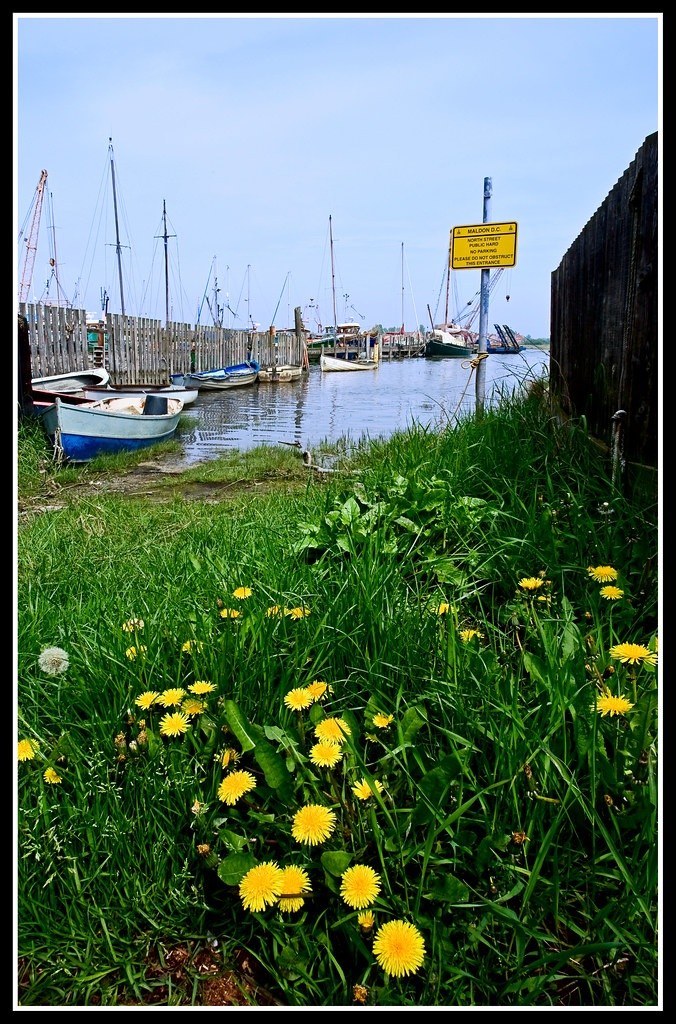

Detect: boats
[80, 381, 199, 408]
[31, 368, 110, 408]
[41, 394, 185, 467]
[183, 358, 260, 391]
[319, 354, 379, 371]
[258, 363, 302, 381]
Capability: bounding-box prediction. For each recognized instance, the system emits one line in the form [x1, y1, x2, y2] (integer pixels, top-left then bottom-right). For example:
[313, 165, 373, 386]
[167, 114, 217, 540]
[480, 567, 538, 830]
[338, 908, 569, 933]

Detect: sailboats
[18, 138, 528, 356]
